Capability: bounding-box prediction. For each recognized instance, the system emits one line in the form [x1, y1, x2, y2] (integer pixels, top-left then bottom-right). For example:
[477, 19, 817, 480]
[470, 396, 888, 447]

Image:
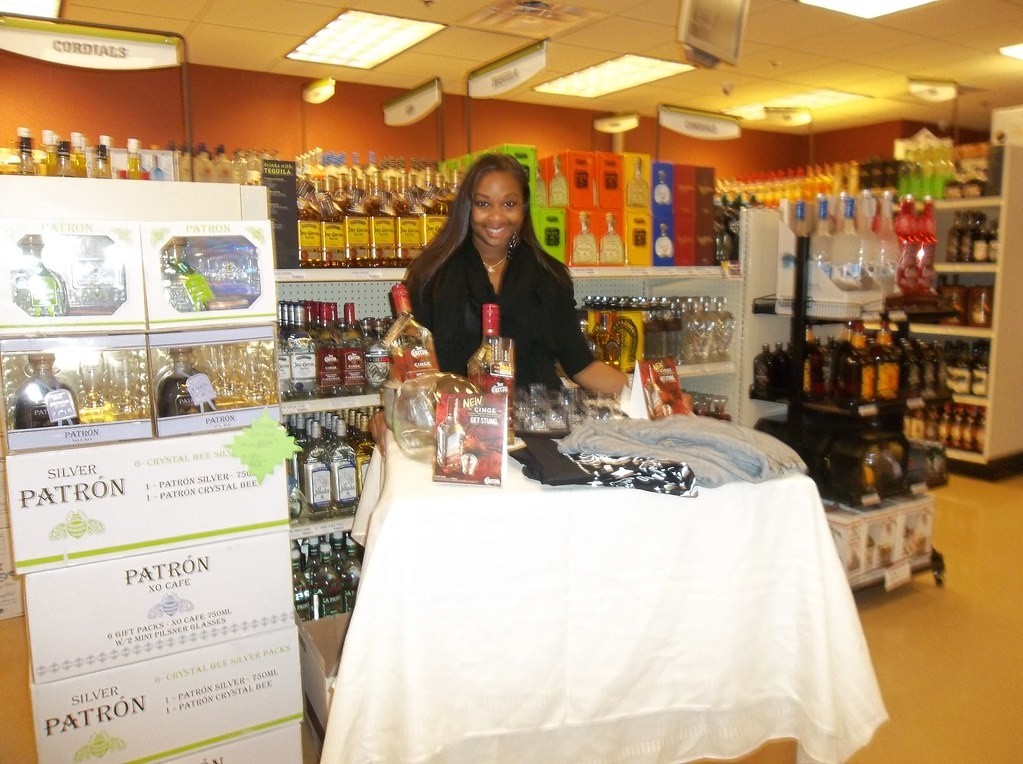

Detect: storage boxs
[436, 143, 720, 267]
[0, 190, 354, 764]
[817, 491, 943, 588]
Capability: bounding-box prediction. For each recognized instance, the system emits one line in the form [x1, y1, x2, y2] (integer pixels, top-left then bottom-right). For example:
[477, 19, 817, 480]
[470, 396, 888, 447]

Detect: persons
[368, 154, 692, 457]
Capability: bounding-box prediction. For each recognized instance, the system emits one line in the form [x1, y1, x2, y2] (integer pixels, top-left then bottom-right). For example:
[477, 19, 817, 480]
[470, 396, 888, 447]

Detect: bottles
[276, 297, 396, 402]
[13, 351, 79, 429]
[289, 531, 364, 622]
[0, 126, 266, 187]
[384, 283, 441, 386]
[153, 347, 216, 417]
[579, 292, 737, 421]
[293, 146, 464, 268]
[712, 143, 1004, 300]
[752, 310, 991, 499]
[466, 301, 513, 445]
[643, 363, 663, 418]
[534, 153, 675, 266]
[436, 396, 465, 473]
[166, 236, 203, 314]
[282, 405, 385, 524]
[11, 234, 69, 317]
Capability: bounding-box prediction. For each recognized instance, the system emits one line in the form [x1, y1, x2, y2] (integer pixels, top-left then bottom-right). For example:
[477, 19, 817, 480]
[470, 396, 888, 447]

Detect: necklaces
[483, 255, 507, 271]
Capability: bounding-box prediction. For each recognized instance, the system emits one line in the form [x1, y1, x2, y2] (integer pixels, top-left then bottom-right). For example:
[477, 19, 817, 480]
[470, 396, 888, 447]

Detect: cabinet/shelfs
[709, 132, 1019, 463]
[282, 267, 750, 619]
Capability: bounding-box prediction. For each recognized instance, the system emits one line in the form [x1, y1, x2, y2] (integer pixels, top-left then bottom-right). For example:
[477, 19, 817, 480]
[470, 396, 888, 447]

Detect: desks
[315, 423, 890, 763]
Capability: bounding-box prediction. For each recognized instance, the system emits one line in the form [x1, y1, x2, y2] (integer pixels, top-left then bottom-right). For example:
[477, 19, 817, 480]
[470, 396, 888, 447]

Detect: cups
[79, 349, 151, 418]
[201, 340, 276, 406]
[381, 377, 398, 430]
[514, 384, 620, 433]
[661, 402, 674, 417]
[462, 453, 478, 476]
[195, 243, 263, 298]
